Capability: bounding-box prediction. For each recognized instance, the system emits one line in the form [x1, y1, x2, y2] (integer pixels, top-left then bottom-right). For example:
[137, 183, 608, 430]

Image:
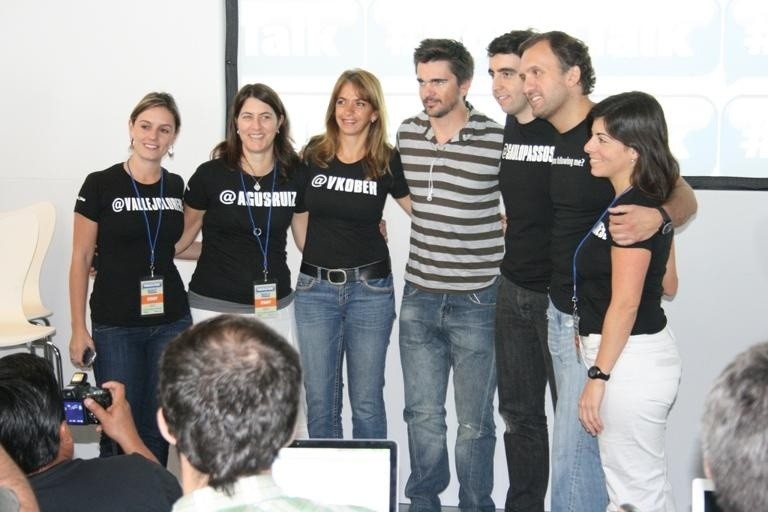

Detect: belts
[299, 259, 390, 286]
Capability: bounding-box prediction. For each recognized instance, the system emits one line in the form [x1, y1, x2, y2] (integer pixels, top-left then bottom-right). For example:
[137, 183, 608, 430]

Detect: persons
[487, 30, 564, 511]
[518, 31, 697, 512]
[0, 445, 40, 511]
[294, 69, 412, 439]
[85, 84, 387, 437]
[151, 314, 371, 512]
[701, 343, 767, 512]
[573, 92, 683, 512]
[392, 37, 506, 511]
[1, 353, 184, 511]
[69, 91, 204, 470]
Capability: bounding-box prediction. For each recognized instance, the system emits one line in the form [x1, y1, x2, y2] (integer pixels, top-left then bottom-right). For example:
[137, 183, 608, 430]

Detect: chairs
[1, 208, 64, 392]
[0, 201, 57, 365]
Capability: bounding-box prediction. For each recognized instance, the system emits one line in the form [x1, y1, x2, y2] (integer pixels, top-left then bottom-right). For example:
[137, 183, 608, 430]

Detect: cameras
[61, 373, 112, 425]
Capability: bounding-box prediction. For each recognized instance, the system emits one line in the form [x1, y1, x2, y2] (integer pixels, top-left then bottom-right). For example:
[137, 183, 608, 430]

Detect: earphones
[427, 191, 432, 201]
[430, 187, 434, 196]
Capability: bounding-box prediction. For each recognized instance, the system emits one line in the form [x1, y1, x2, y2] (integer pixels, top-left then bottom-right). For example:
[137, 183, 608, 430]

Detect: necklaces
[240, 154, 276, 191]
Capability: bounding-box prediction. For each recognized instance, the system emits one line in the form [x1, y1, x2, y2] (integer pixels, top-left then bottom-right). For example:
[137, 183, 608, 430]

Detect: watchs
[657, 205, 674, 235]
[586, 365, 610, 381]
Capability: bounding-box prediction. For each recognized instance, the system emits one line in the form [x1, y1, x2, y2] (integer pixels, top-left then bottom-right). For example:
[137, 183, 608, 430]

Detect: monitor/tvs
[692, 478, 724, 512]
[271, 440, 397, 512]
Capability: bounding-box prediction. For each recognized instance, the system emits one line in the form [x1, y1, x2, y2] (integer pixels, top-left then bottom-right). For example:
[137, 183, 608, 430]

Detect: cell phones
[83, 348, 96, 366]
